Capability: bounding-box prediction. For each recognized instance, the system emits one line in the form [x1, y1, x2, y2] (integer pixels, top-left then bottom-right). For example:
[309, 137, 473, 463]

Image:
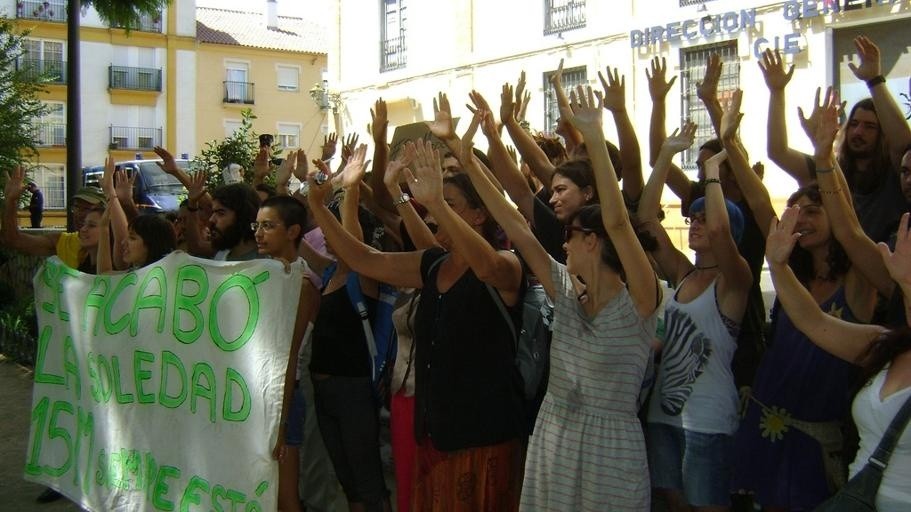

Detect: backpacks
[428, 251, 549, 401]
[321, 261, 401, 386]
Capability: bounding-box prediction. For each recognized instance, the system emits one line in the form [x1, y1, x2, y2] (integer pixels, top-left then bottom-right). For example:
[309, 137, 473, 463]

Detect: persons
[0, 35, 911, 512]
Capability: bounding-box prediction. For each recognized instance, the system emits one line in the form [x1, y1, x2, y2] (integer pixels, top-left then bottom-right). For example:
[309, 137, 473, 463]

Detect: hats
[71, 187, 107, 206]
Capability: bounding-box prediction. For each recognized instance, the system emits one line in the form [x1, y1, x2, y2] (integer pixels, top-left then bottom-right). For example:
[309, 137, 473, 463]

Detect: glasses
[685, 215, 706, 225]
[250, 222, 285, 231]
[562, 225, 593, 242]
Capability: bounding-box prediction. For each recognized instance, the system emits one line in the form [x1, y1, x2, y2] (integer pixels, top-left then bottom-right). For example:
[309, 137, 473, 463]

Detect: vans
[81, 151, 202, 219]
